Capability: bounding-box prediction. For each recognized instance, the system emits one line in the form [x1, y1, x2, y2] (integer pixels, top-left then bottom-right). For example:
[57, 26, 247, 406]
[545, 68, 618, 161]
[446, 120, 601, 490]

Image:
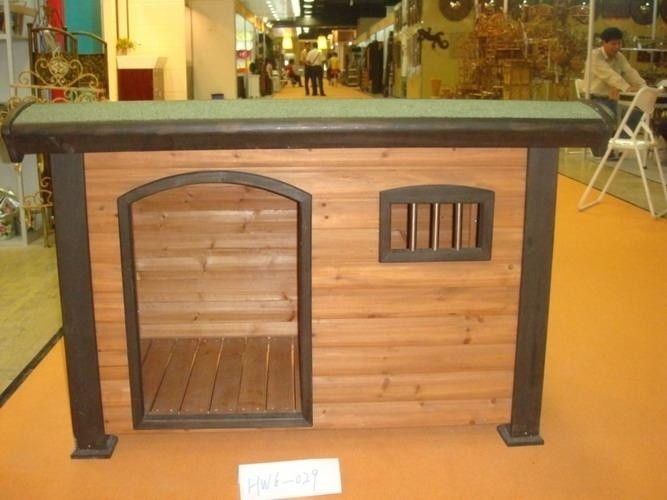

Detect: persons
[287, 41, 340, 96]
[585, 28, 649, 161]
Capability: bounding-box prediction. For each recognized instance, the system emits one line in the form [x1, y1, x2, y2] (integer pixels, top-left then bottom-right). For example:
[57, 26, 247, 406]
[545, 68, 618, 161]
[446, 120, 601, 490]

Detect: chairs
[564, 78, 667, 219]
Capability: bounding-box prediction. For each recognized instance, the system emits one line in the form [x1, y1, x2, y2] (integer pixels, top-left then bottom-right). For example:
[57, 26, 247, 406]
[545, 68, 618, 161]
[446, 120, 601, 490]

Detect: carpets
[0, 225, 65, 406]
[553, 145, 667, 220]
